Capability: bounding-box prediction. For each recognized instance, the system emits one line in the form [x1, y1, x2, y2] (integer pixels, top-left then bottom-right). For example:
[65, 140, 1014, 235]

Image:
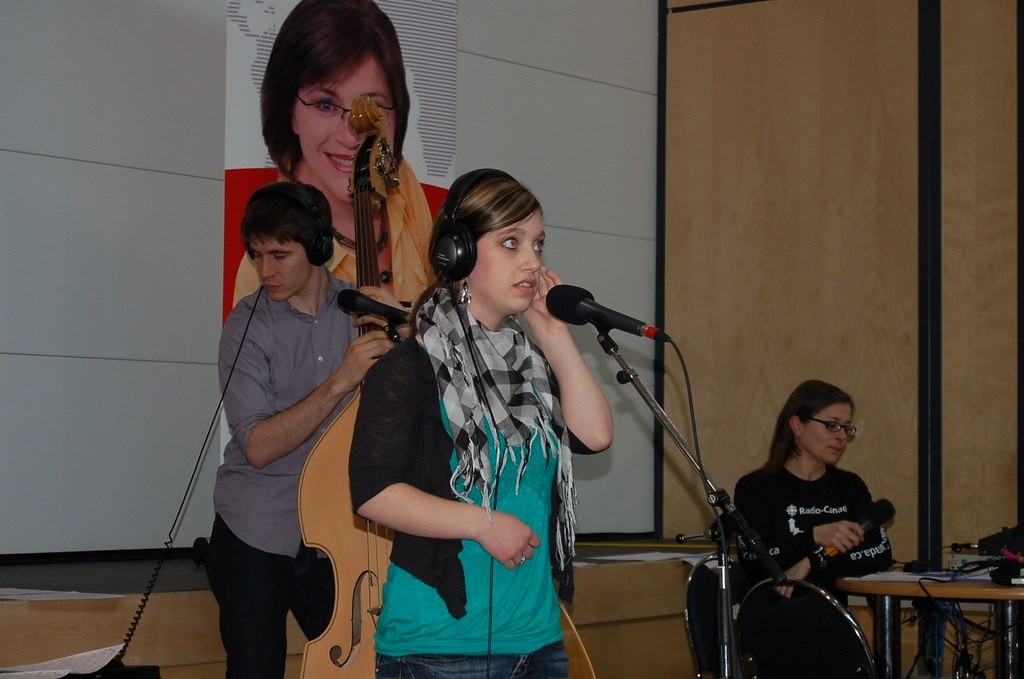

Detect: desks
[839, 567, 1023, 679]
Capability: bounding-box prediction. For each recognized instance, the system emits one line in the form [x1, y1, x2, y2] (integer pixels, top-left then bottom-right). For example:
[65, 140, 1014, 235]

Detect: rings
[519, 556, 525, 565]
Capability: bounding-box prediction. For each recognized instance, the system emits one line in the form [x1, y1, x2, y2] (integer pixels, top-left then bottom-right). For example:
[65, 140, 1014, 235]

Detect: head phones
[245, 181, 334, 267]
[430, 169, 516, 282]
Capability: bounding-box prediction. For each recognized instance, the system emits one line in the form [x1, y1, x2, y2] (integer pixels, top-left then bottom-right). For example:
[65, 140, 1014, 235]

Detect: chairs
[687, 554, 745, 679]
[733, 577, 879, 678]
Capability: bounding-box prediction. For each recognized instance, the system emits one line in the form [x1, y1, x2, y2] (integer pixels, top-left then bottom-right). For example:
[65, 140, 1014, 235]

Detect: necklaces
[332, 229, 386, 249]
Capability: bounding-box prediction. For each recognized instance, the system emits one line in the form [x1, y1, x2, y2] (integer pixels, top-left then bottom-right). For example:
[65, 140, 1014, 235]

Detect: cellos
[298, 95, 599, 679]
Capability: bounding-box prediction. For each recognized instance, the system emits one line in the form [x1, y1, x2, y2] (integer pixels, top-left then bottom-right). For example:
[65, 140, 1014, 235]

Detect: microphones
[821, 499, 896, 559]
[546, 284, 672, 343]
[338, 289, 414, 324]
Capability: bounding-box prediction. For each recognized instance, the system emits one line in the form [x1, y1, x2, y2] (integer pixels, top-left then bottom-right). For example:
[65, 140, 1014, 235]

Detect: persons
[348, 167, 616, 679]
[208, 181, 410, 679]
[734, 379, 892, 679]
[221, 0, 450, 333]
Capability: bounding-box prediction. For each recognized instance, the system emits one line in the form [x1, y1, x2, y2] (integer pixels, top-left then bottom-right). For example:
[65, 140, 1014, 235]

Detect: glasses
[803, 416, 857, 437]
[296, 95, 394, 128]
